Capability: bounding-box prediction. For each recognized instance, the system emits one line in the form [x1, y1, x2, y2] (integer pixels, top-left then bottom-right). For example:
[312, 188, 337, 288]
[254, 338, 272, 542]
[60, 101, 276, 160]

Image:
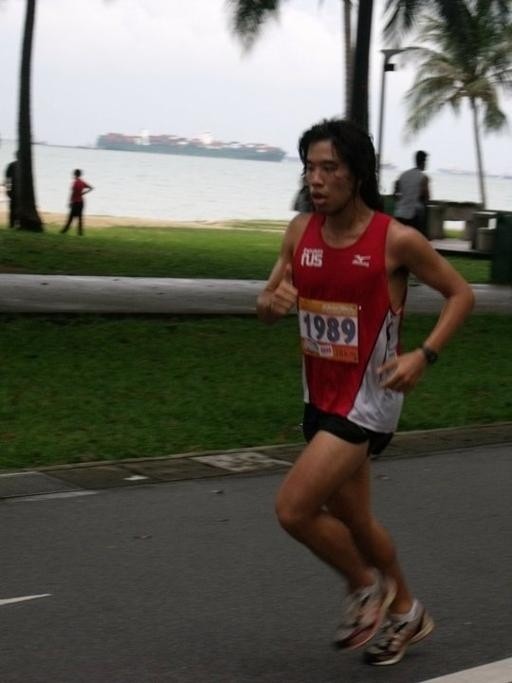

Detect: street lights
[373, 44, 416, 201]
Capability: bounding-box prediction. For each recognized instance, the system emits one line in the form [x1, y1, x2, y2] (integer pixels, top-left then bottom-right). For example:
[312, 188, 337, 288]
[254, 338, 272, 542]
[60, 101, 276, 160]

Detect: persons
[392, 149, 430, 240]
[60, 168, 95, 236]
[253, 114, 475, 668]
[4, 149, 23, 229]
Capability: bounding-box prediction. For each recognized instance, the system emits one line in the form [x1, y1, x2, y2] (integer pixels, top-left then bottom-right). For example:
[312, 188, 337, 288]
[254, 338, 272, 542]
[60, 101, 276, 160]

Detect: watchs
[416, 343, 438, 364]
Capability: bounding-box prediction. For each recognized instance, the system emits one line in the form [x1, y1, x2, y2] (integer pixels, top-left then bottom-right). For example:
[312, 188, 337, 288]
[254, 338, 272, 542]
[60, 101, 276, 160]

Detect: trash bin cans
[491, 211, 512, 283]
[426, 204, 443, 238]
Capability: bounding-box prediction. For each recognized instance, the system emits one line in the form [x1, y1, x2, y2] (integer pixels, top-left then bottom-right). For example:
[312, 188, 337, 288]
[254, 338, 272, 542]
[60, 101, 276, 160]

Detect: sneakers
[330, 575, 436, 666]
